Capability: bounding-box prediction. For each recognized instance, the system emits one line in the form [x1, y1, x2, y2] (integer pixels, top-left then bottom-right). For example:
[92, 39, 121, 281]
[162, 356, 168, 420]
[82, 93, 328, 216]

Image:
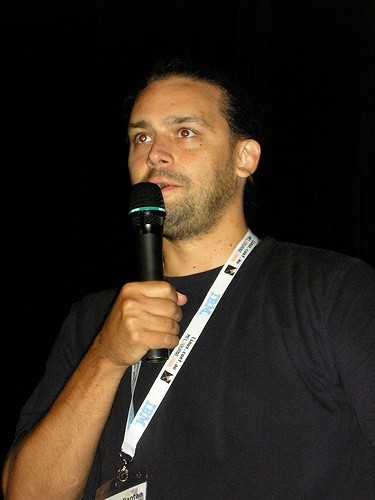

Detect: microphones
[127, 182, 171, 365]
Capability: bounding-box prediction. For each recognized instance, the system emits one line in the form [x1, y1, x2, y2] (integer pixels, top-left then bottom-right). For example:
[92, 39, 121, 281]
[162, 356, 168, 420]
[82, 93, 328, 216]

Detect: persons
[0, 60, 375, 500]
